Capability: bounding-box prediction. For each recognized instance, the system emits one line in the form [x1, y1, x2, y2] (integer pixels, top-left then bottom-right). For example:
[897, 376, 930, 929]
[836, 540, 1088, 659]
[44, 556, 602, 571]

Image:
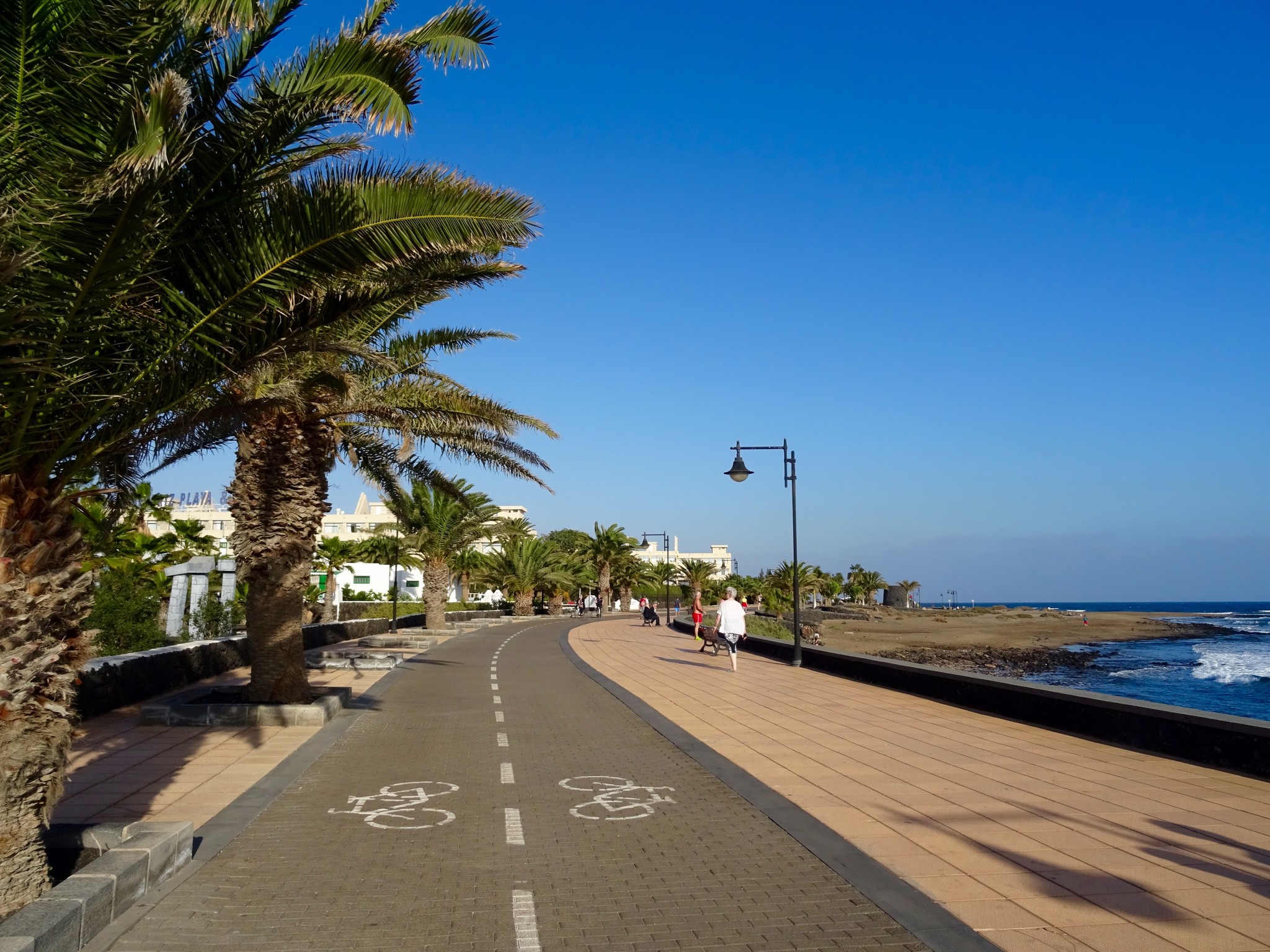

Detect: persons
[909, 594, 913, 602]
[576, 594, 585, 615]
[741, 595, 748, 613]
[720, 598, 725, 602]
[757, 592, 762, 610]
[713, 586, 747, 672]
[1083, 615, 1088, 626]
[595, 594, 604, 617]
[692, 591, 706, 640]
[813, 633, 819, 643]
[639, 595, 650, 619]
[646, 603, 661, 627]
[674, 596, 681, 616]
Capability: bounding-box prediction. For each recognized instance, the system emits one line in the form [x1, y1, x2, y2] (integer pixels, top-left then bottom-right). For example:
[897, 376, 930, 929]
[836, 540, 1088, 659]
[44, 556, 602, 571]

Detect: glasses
[655, 606, 657, 607]
[725, 592, 727, 594]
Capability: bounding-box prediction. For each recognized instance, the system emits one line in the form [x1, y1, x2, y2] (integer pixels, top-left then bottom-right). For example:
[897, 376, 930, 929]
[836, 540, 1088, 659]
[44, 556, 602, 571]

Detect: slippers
[726, 668, 736, 673]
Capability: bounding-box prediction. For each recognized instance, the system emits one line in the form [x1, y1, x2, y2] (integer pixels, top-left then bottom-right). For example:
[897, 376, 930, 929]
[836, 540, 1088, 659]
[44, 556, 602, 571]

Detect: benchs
[686, 609, 693, 615]
[699, 628, 729, 657]
[641, 613, 653, 627]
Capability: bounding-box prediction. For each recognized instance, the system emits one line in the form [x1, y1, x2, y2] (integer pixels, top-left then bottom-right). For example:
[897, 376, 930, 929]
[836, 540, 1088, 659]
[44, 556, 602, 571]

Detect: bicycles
[596, 602, 602, 618]
[575, 602, 585, 619]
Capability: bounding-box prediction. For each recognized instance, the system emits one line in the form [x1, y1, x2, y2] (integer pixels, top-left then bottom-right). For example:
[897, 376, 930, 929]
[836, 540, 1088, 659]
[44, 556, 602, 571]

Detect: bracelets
[713, 626, 717, 629]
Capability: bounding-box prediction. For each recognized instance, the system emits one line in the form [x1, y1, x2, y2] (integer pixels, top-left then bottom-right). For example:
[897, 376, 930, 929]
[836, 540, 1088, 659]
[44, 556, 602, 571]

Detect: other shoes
[694, 636, 702, 640]
[655, 624, 661, 627]
[640, 617, 644, 619]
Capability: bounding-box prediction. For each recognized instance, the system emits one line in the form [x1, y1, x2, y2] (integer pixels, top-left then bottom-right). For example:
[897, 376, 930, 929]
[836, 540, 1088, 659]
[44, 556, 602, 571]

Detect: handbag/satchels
[641, 599, 645, 606]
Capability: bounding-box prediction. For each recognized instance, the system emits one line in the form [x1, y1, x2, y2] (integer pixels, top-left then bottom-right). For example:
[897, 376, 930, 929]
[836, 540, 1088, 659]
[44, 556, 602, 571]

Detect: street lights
[723, 440, 803, 668]
[940, 593, 943, 609]
[947, 589, 958, 609]
[914, 586, 920, 608]
[720, 557, 739, 603]
[640, 531, 671, 625]
